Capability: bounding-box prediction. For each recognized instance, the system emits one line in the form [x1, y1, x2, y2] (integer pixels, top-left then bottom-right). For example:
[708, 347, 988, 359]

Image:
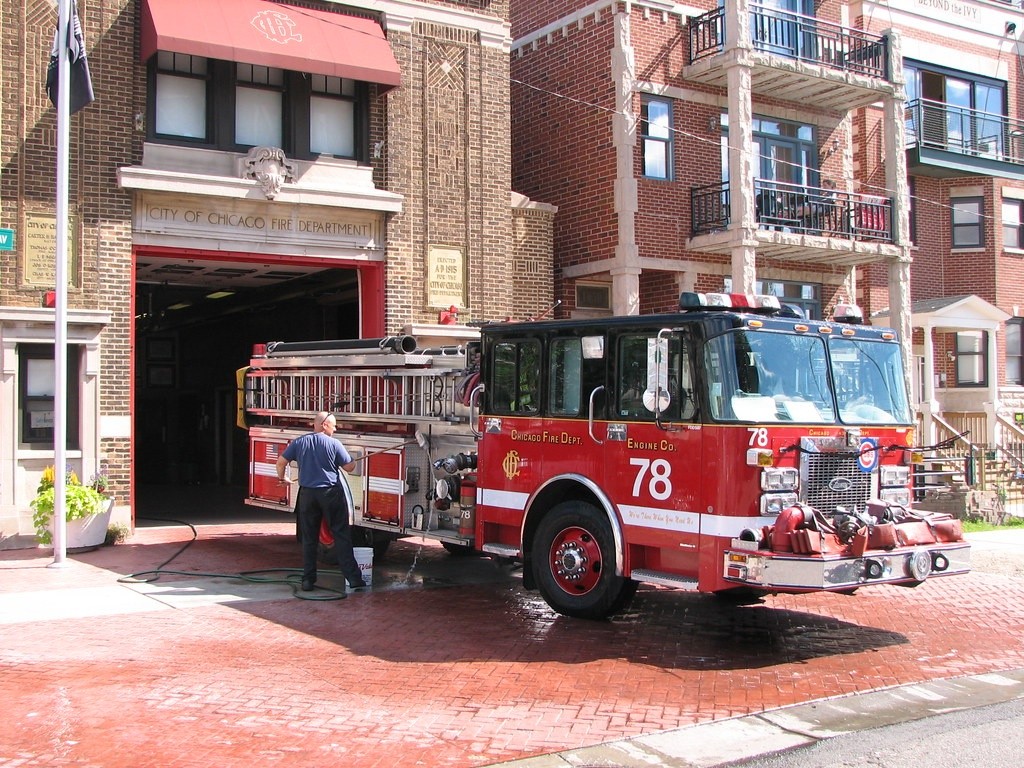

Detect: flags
[45, 0, 94, 115]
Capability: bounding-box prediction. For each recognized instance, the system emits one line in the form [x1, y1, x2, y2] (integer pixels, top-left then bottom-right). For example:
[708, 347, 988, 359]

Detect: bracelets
[279, 478, 286, 481]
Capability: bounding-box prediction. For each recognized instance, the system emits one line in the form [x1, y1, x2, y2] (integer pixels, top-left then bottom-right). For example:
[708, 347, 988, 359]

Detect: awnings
[140, 0, 401, 97]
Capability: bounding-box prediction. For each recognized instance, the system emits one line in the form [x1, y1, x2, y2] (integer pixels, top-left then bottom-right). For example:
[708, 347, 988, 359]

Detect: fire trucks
[236, 293, 971, 618]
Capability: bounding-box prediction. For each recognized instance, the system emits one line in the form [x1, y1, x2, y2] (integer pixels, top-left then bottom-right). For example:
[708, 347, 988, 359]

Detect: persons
[798, 179, 836, 216]
[276, 412, 366, 592]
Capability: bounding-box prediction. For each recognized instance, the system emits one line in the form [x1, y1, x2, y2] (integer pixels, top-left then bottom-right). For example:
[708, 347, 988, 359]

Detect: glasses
[320, 412, 333, 427]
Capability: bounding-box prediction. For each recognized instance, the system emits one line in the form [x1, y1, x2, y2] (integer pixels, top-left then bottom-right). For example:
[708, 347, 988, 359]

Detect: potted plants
[31, 485, 115, 556]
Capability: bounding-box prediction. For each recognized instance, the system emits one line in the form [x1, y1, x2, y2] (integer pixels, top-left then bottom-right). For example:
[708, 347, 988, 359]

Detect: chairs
[756, 194, 788, 232]
[737, 364, 759, 393]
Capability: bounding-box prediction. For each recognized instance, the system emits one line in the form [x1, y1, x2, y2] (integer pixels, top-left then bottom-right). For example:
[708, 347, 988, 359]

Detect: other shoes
[302, 580, 313, 591]
[350, 580, 366, 588]
